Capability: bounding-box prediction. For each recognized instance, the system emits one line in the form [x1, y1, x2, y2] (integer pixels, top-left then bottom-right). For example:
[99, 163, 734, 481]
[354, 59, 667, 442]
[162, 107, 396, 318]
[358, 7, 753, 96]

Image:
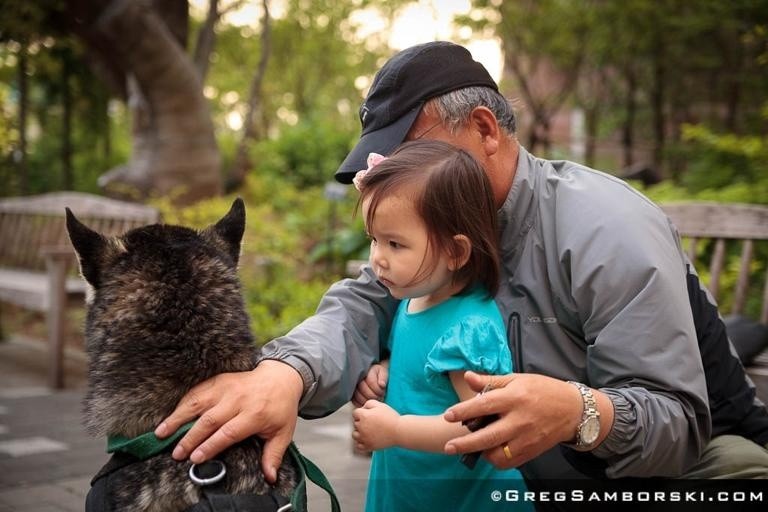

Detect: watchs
[565, 379, 601, 453]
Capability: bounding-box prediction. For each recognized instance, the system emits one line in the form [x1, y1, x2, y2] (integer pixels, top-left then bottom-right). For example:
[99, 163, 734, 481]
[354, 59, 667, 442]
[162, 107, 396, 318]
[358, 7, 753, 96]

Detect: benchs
[659, 204, 768, 404]
[0, 193, 164, 391]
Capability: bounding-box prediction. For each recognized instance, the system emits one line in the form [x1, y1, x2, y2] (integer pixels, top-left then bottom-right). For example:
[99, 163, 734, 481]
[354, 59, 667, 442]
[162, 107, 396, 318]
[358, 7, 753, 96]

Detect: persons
[348, 136, 539, 511]
[153, 37, 766, 508]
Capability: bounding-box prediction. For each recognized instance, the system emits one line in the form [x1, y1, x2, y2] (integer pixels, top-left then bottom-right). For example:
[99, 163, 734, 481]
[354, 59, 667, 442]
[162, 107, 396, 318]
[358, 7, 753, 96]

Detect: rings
[503, 445, 512, 461]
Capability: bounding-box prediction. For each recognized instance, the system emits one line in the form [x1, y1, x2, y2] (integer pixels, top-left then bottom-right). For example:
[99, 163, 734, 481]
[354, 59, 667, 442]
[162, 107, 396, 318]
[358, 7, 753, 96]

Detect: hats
[335, 42, 498, 184]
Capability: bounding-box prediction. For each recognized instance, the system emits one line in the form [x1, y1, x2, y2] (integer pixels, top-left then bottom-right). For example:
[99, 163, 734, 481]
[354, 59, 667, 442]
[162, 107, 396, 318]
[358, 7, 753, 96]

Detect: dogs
[64, 196, 307, 511]
